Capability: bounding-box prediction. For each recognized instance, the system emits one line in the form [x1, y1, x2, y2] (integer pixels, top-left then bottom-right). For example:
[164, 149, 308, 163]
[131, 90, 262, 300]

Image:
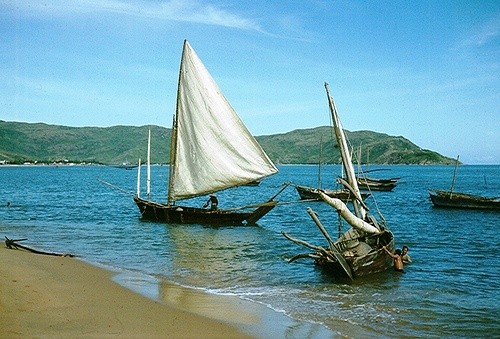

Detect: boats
[428, 190, 499, 211]
[291, 183, 371, 203]
[335, 177, 399, 192]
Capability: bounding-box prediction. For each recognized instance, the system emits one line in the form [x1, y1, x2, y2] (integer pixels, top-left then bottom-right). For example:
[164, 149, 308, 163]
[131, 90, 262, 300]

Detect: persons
[204, 194, 218, 210]
[383, 246, 412, 272]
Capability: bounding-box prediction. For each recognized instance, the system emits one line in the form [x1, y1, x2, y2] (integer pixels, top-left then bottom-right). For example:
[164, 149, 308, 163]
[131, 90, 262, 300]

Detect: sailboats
[96, 39, 293, 226]
[315, 81, 394, 278]
